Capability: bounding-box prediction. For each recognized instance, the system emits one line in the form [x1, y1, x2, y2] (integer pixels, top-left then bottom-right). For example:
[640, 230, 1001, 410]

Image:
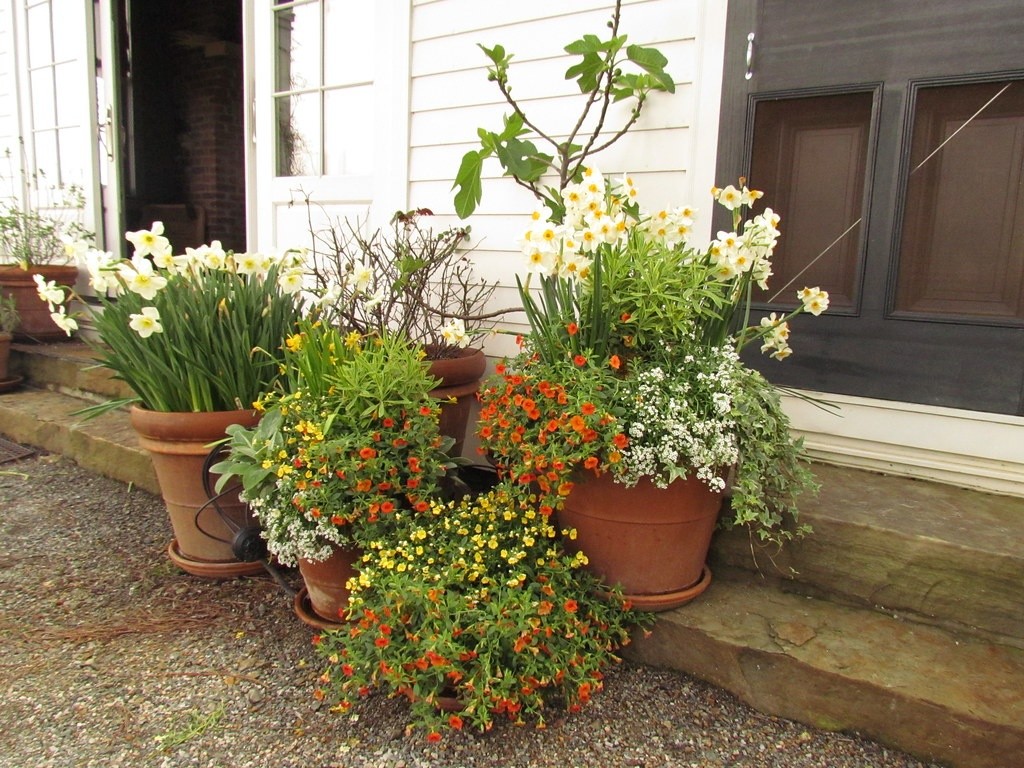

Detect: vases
[128, 403, 268, 578]
[557, 451, 732, 615]
[430, 384, 484, 456]
[0, 191, 97, 385]
[399, 679, 469, 716]
[32, 221, 470, 415]
[295, 543, 374, 631]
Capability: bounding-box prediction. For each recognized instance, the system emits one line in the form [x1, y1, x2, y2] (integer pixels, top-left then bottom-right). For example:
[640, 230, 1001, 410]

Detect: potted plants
[294, 183, 500, 384]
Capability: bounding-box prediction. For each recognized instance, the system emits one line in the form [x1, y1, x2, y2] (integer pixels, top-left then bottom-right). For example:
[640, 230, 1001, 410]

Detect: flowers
[473, 161, 830, 583]
[306, 481, 656, 742]
[203, 315, 473, 570]
